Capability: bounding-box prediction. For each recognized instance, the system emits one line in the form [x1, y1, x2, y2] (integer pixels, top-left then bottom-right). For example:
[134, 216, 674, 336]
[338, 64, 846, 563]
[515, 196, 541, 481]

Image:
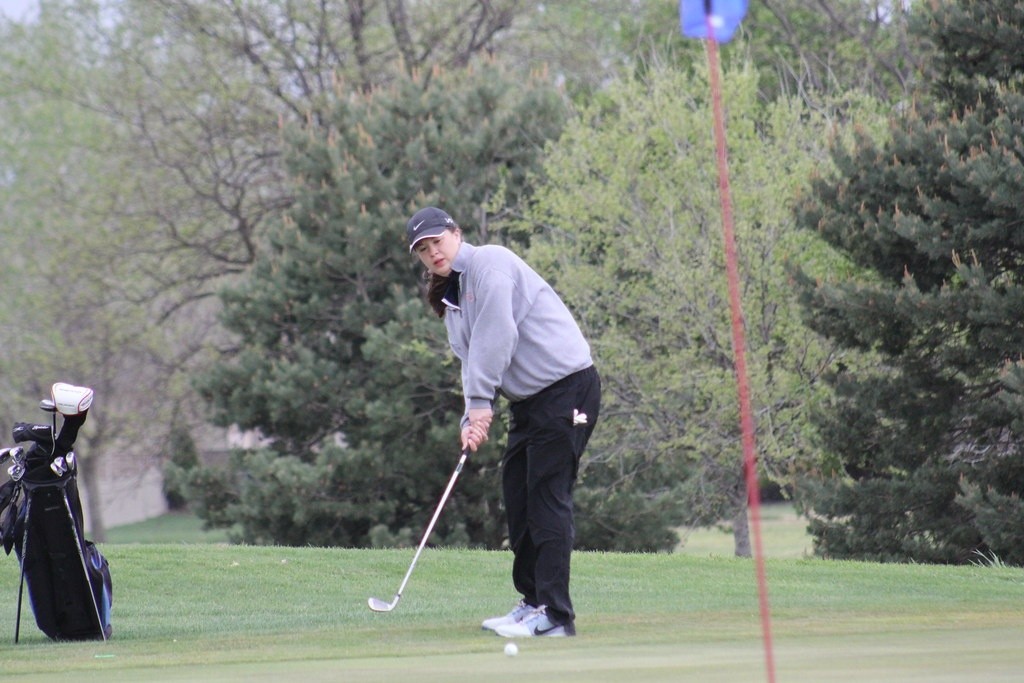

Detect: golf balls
[503, 643, 518, 657]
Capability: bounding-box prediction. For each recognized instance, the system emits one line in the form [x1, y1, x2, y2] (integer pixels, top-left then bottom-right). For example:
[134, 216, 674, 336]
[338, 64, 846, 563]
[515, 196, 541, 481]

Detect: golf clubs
[368, 443, 471, 612]
[1, 381, 113, 641]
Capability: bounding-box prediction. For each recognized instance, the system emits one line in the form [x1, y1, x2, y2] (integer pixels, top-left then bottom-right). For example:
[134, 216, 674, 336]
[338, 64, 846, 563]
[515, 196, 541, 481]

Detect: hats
[407, 208, 456, 254]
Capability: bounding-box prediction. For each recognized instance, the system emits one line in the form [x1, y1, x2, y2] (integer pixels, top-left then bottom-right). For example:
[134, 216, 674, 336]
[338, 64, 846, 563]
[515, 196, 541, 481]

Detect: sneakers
[481, 598, 538, 631]
[496, 604, 575, 637]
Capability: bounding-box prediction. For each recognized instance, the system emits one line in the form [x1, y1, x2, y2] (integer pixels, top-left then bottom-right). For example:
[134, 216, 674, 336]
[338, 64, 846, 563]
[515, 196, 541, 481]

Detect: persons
[406, 206, 602, 637]
